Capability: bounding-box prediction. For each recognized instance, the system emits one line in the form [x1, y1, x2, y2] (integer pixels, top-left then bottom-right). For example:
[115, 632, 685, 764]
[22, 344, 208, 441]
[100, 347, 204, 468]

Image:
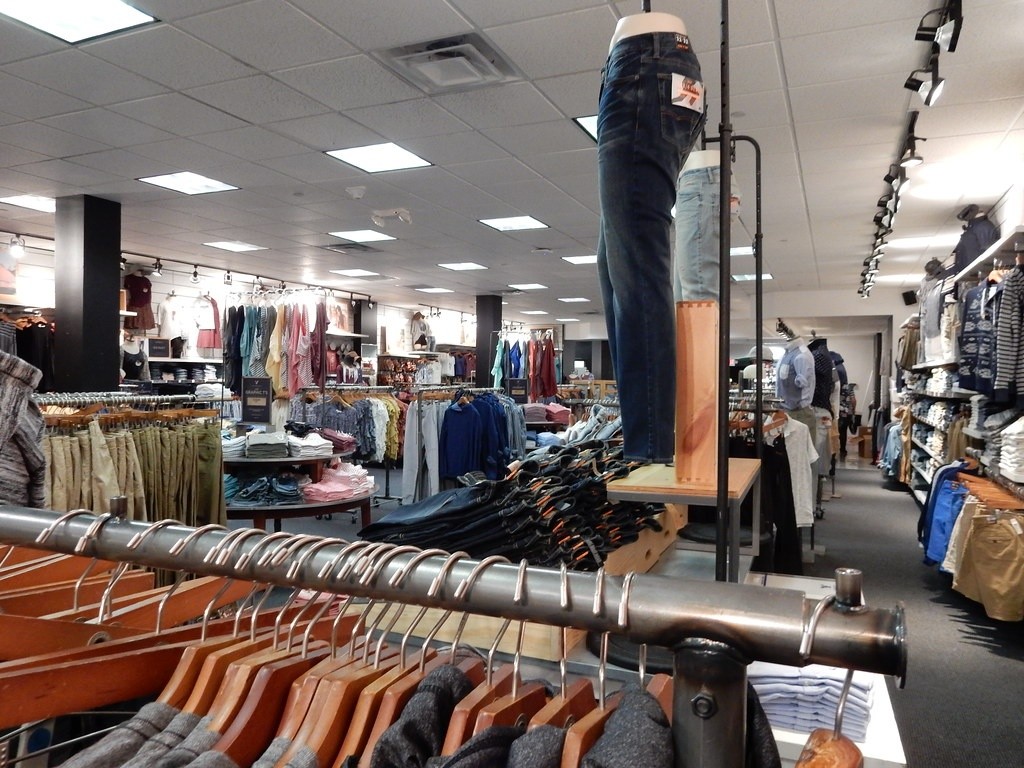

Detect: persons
[120, 336, 151, 382]
[157, 294, 189, 358]
[776, 336, 848, 462]
[193, 293, 223, 349]
[916, 257, 943, 363]
[411, 312, 432, 349]
[124, 270, 155, 329]
[950, 212, 1000, 274]
[597, 12, 737, 464]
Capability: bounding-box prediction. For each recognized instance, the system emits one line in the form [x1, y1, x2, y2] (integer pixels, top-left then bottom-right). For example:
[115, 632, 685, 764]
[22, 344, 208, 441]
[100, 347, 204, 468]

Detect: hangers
[726, 394, 790, 442]
[980, 240, 1024, 287]
[0, 307, 48, 330]
[448, 351, 477, 359]
[33, 392, 221, 438]
[497, 330, 553, 342]
[0, 507, 675, 768]
[794, 593, 866, 768]
[552, 385, 621, 422]
[300, 383, 508, 409]
[223, 285, 336, 308]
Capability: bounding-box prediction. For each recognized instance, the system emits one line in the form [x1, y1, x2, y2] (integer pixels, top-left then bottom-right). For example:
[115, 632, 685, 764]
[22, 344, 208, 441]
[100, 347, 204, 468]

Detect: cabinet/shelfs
[901, 353, 992, 515]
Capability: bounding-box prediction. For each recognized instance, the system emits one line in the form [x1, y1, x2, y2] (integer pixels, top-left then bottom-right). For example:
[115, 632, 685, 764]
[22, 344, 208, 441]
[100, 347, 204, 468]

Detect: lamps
[350, 293, 355, 308]
[859, 0, 965, 299]
[120, 251, 262, 289]
[368, 296, 373, 309]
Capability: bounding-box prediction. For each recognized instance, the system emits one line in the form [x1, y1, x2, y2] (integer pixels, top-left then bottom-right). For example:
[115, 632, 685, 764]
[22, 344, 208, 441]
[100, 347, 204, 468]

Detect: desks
[603, 454, 762, 585]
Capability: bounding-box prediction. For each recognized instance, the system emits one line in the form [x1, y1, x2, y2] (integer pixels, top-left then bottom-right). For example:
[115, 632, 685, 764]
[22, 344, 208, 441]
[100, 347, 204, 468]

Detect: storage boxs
[849, 426, 875, 458]
